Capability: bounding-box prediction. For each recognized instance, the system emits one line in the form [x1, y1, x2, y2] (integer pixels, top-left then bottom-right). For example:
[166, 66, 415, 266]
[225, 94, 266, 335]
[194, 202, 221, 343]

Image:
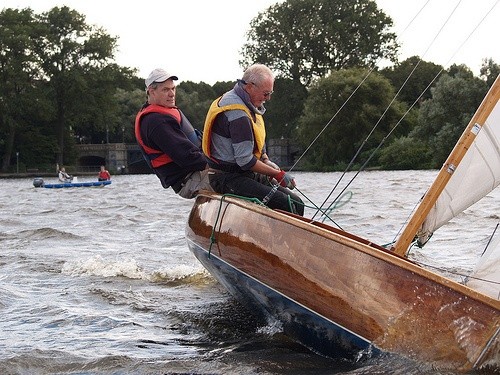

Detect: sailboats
[181, 0, 500, 375]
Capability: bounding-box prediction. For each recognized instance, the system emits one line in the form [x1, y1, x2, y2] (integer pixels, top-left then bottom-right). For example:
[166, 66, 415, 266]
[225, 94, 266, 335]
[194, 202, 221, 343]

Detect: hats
[145, 68, 179, 88]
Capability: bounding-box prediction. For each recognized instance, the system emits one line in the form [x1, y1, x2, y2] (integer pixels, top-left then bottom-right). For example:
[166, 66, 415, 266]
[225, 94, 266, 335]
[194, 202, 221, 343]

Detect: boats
[33, 178, 112, 188]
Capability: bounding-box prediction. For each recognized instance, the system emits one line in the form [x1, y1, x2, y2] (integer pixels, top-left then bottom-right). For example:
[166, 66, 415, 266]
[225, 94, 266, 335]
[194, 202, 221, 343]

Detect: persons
[203, 64, 304, 215]
[135, 69, 215, 199]
[59, 167, 70, 183]
[98, 166, 110, 181]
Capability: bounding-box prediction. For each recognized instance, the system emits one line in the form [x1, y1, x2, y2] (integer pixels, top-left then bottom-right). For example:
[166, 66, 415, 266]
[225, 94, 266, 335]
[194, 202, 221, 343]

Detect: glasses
[252, 82, 274, 97]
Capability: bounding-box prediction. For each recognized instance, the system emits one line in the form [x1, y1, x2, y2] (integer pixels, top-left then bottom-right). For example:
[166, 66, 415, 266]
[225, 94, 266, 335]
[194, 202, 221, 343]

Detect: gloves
[275, 170, 296, 191]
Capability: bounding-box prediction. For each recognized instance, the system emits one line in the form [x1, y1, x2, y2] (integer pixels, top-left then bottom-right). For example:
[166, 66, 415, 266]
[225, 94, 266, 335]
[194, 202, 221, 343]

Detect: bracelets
[262, 154, 267, 161]
